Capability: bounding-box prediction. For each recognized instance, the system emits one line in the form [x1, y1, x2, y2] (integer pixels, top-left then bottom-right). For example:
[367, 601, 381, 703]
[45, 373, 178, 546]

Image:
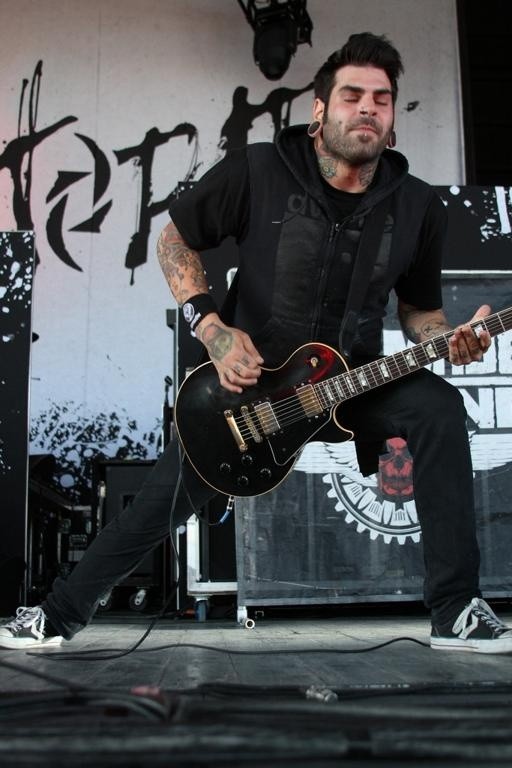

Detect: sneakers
[429, 598, 512, 655]
[0, 605, 63, 649]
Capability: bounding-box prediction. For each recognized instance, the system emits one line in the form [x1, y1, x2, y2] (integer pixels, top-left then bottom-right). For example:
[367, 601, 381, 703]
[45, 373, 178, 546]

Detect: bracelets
[180, 291, 221, 332]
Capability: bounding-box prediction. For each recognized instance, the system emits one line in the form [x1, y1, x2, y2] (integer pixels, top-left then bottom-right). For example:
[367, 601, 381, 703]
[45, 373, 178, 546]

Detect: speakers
[0, 230, 35, 617]
[175, 184, 512, 269]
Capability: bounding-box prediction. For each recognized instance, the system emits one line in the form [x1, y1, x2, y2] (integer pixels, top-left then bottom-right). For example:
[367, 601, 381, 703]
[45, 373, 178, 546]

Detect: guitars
[174, 309, 511, 495]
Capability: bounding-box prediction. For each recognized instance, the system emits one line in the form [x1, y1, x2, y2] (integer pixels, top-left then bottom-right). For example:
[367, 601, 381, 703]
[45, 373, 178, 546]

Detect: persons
[0, 28, 512, 657]
